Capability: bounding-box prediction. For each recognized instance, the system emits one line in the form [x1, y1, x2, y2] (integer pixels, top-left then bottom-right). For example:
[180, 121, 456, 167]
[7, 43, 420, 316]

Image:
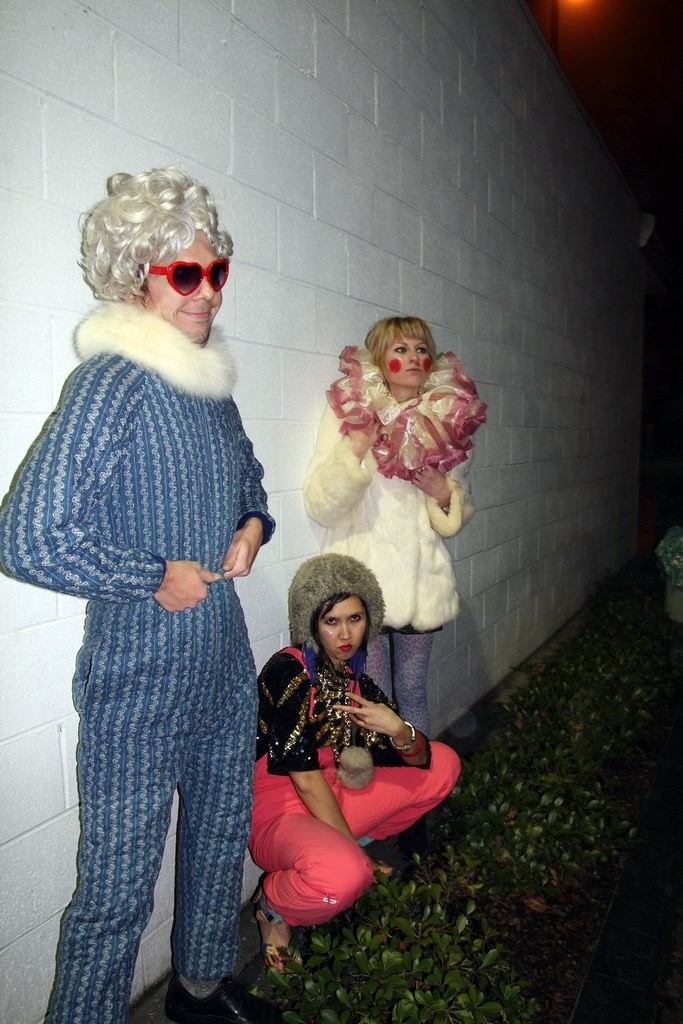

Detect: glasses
[142, 258, 230, 295]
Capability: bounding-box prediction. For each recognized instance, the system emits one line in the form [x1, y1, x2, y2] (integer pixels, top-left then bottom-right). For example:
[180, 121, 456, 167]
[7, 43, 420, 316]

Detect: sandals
[252, 887, 306, 973]
[371, 855, 404, 883]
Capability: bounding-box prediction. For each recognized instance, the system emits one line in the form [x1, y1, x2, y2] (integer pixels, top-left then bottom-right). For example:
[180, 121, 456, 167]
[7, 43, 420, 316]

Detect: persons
[249, 553, 461, 975]
[301, 317, 489, 860]
[0, 168, 275, 1024]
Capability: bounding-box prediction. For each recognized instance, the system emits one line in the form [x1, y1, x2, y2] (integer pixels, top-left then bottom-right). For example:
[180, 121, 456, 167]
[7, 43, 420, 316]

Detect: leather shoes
[163, 970, 287, 1024]
[397, 823, 428, 858]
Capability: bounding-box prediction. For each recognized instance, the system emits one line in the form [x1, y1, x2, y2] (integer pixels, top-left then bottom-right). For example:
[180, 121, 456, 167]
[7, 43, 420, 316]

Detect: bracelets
[441, 506, 449, 514]
[390, 721, 415, 751]
[396, 730, 424, 757]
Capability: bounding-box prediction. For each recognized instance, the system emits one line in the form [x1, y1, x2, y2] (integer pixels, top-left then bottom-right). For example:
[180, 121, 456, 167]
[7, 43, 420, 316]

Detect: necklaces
[319, 665, 351, 761]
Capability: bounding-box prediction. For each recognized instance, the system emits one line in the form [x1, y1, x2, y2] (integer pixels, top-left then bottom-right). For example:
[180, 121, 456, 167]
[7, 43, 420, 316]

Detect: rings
[421, 466, 425, 471]
